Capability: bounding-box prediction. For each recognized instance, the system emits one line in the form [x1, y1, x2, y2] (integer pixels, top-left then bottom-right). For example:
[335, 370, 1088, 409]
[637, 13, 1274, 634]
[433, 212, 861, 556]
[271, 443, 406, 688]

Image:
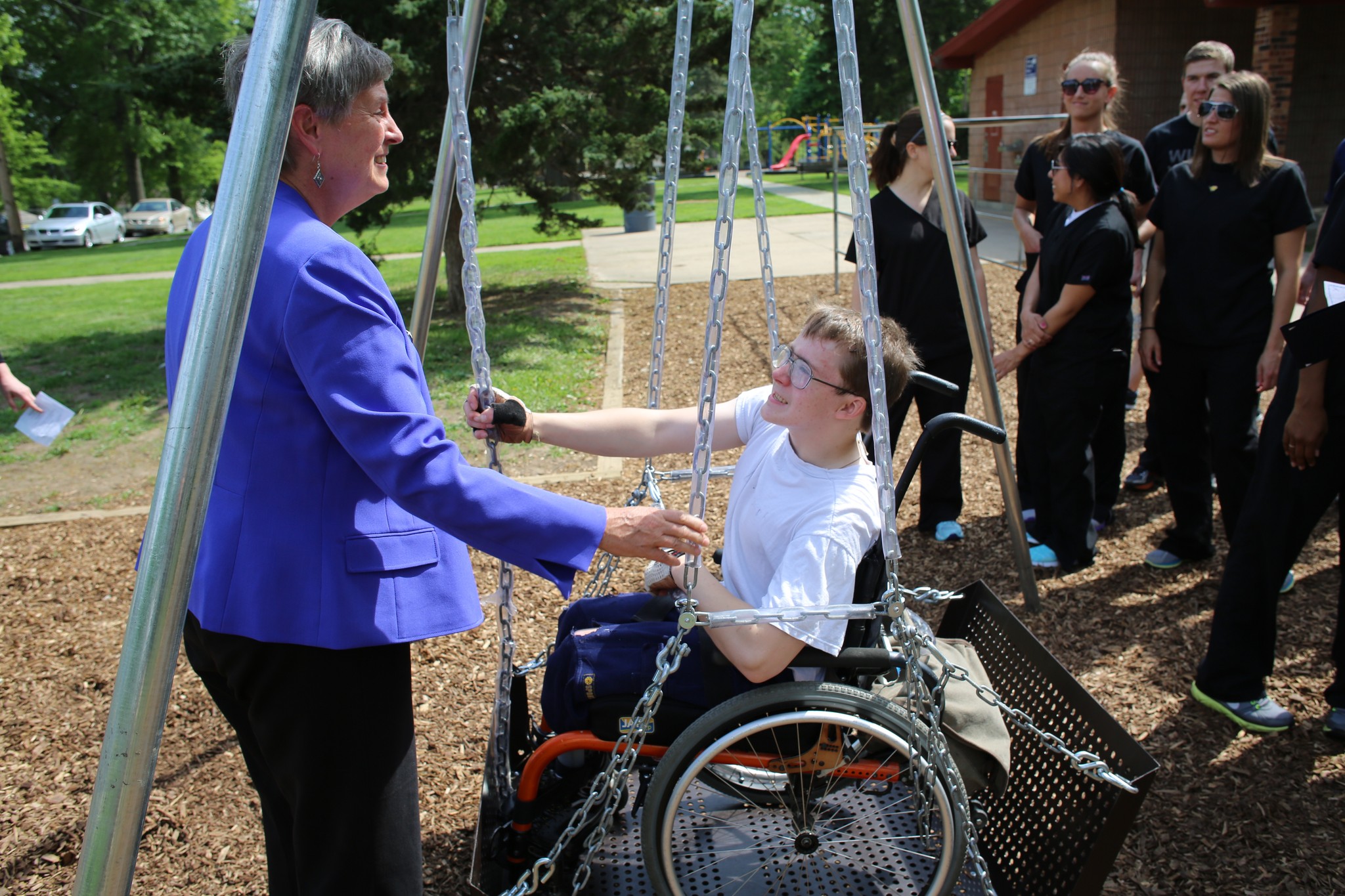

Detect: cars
[121, 197, 195, 238]
[22, 201, 126, 251]
[0, 214, 23, 257]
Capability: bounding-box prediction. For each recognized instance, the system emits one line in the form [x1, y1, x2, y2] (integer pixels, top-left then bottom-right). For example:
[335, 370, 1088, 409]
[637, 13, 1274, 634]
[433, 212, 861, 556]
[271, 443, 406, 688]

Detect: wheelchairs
[464, 363, 1007, 896]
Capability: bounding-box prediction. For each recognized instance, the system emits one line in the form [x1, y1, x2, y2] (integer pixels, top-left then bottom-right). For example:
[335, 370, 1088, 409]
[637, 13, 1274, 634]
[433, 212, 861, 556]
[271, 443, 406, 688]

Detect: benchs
[797, 161, 847, 180]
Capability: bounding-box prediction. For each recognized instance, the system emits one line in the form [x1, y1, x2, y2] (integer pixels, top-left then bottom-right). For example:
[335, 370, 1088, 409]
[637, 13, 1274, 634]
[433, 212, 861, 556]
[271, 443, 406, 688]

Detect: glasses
[772, 344, 857, 398]
[947, 140, 959, 150]
[1061, 77, 1110, 95]
[1051, 159, 1069, 176]
[1197, 99, 1241, 121]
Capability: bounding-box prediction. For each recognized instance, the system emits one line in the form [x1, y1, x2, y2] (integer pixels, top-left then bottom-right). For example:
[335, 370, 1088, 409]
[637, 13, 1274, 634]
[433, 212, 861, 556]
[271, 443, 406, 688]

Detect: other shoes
[1029, 544, 1060, 567]
[1124, 466, 1166, 491]
[1144, 538, 1215, 569]
[1125, 389, 1138, 410]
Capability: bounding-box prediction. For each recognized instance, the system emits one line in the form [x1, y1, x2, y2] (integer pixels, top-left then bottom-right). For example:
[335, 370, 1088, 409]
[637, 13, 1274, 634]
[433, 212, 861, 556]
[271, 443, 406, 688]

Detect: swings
[442, 0, 1164, 896]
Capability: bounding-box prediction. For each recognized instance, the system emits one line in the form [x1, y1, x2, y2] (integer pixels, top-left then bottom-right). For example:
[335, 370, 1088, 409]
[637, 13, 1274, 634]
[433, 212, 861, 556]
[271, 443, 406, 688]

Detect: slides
[770, 133, 811, 170]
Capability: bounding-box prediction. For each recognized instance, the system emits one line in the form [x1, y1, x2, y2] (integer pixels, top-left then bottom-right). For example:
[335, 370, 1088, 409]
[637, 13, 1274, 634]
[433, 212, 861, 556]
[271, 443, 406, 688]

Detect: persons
[0, 352, 43, 415]
[1191, 174, 1345, 734]
[994, 40, 1345, 593]
[844, 106, 987, 545]
[135, 20, 711, 896]
[462, 303, 923, 896]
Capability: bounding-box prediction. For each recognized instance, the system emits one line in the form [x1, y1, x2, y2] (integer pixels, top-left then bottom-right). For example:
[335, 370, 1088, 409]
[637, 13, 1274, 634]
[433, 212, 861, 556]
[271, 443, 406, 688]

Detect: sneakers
[935, 519, 964, 541]
[1191, 677, 1294, 733]
[1323, 707, 1345, 738]
